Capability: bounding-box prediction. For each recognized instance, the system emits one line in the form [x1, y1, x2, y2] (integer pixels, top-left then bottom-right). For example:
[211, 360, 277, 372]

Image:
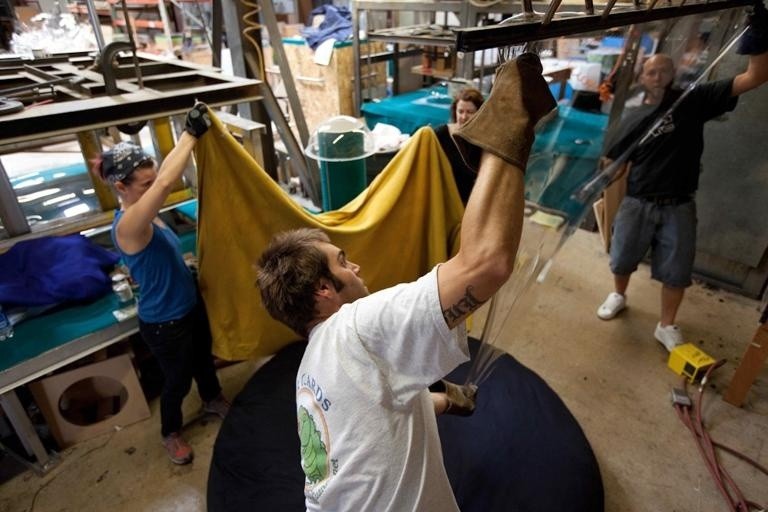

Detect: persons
[433, 89, 489, 213]
[90, 100, 233, 469]
[253, 52, 543, 511]
[594, 0, 768, 362]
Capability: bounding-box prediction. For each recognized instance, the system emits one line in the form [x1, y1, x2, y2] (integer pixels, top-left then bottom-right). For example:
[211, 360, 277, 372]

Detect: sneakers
[595, 291, 628, 321]
[202, 394, 237, 420]
[652, 319, 683, 353]
[159, 430, 195, 466]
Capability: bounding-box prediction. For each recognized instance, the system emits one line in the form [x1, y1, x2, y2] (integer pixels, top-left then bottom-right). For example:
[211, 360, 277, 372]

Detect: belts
[636, 194, 694, 207]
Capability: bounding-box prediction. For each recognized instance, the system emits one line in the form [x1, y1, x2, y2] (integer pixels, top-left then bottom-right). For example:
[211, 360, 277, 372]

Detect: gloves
[432, 377, 479, 418]
[450, 49, 560, 175]
[182, 101, 212, 140]
[735, 4, 768, 55]
